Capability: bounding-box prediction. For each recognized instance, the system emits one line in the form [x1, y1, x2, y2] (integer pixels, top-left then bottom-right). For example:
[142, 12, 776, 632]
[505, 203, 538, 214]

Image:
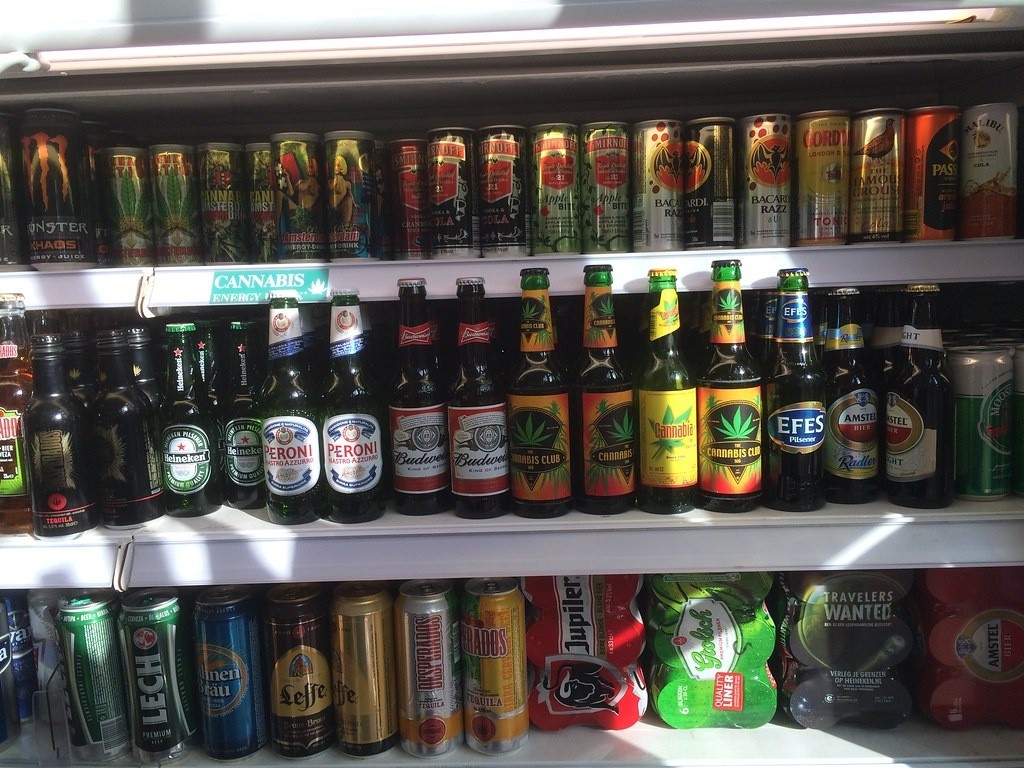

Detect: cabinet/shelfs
[0, 5, 1024, 768]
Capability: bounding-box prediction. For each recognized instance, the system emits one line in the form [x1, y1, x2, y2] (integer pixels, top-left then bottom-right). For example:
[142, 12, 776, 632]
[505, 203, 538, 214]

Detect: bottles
[0, 285, 387, 540]
[388, 260, 956, 519]
